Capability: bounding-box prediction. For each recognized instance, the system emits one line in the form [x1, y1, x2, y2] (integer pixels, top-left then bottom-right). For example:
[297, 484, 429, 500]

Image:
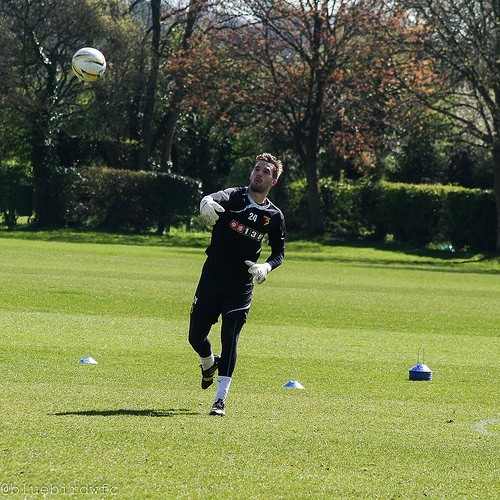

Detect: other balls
[71, 47, 106, 83]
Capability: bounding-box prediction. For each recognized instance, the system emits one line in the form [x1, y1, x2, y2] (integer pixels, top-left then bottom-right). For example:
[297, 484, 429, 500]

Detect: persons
[188, 153, 285, 415]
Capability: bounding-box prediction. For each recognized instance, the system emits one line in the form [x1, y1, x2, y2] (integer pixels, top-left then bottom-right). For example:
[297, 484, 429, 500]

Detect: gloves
[245, 260, 272, 284]
[200, 195, 225, 225]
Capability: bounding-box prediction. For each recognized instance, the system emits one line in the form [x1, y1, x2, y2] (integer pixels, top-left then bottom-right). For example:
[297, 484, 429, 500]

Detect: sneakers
[211, 398, 226, 415]
[199, 355, 221, 390]
